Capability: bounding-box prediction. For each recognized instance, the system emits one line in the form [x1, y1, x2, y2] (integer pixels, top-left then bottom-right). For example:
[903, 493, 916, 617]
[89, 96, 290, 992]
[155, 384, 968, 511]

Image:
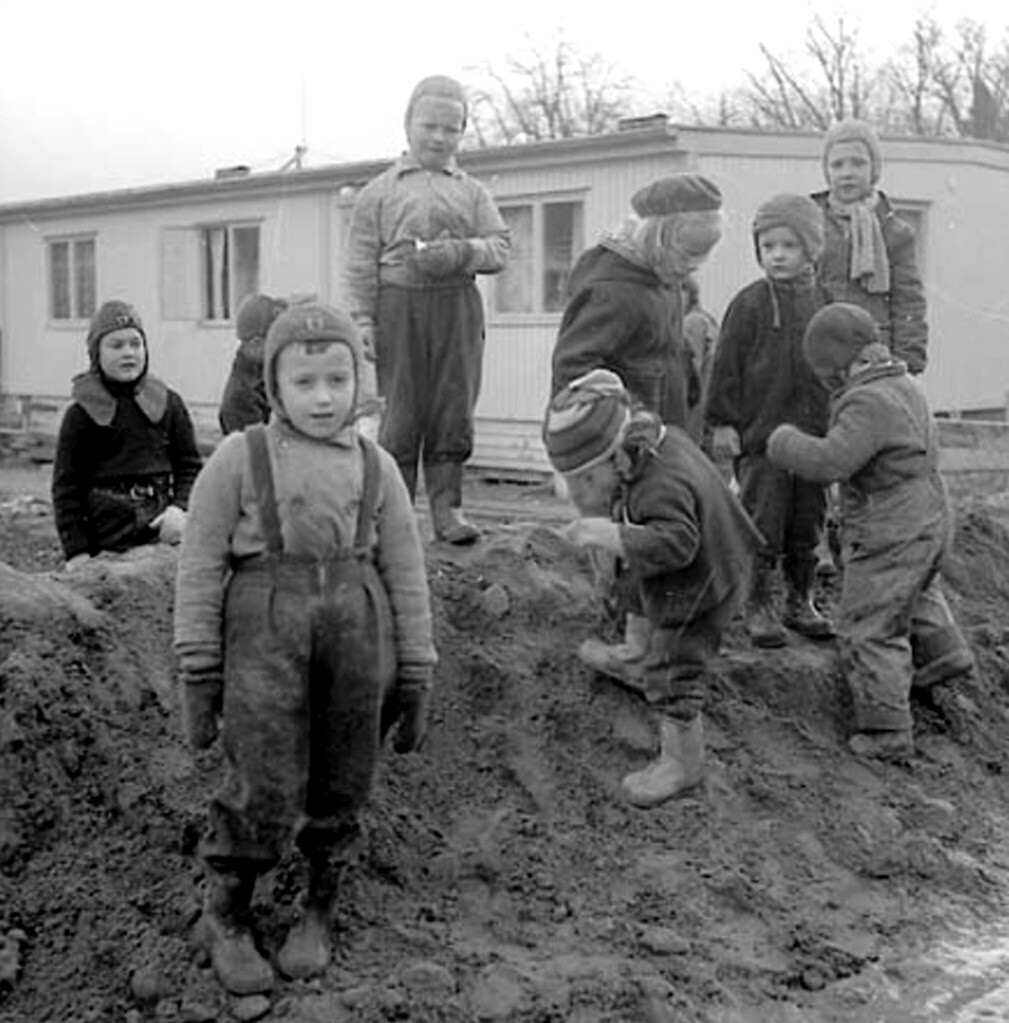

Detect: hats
[263, 294, 365, 427]
[235, 293, 283, 341]
[822, 117, 882, 185]
[803, 302, 879, 388]
[751, 192, 824, 269]
[86, 300, 149, 377]
[630, 173, 722, 219]
[404, 77, 468, 143]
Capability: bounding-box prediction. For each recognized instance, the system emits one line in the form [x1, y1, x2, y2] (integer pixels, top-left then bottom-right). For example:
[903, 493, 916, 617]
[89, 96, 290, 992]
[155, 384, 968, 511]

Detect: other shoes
[278, 895, 340, 977]
[849, 729, 919, 761]
[910, 623, 970, 687]
[198, 910, 275, 993]
[819, 549, 842, 574]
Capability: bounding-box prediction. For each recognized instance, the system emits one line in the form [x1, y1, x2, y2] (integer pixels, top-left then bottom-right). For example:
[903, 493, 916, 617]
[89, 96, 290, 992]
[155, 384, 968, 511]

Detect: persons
[820, 118, 929, 375]
[542, 368, 768, 806]
[680, 274, 718, 374]
[767, 304, 975, 761]
[51, 299, 204, 560]
[705, 192, 832, 644]
[343, 76, 513, 543]
[542, 172, 723, 428]
[218, 293, 289, 437]
[174, 291, 438, 994]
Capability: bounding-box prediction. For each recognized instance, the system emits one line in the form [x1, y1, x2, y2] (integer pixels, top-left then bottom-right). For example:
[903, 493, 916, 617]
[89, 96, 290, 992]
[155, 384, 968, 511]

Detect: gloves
[176, 673, 227, 753]
[741, 428, 773, 453]
[564, 518, 626, 561]
[360, 327, 379, 362]
[148, 504, 184, 546]
[378, 669, 431, 754]
[414, 238, 474, 278]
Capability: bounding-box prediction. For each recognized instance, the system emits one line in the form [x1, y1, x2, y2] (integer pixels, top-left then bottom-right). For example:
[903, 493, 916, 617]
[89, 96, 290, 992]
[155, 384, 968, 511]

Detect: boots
[576, 612, 654, 689]
[621, 714, 704, 806]
[781, 562, 832, 636]
[394, 462, 418, 503]
[423, 459, 480, 544]
[740, 557, 789, 646]
